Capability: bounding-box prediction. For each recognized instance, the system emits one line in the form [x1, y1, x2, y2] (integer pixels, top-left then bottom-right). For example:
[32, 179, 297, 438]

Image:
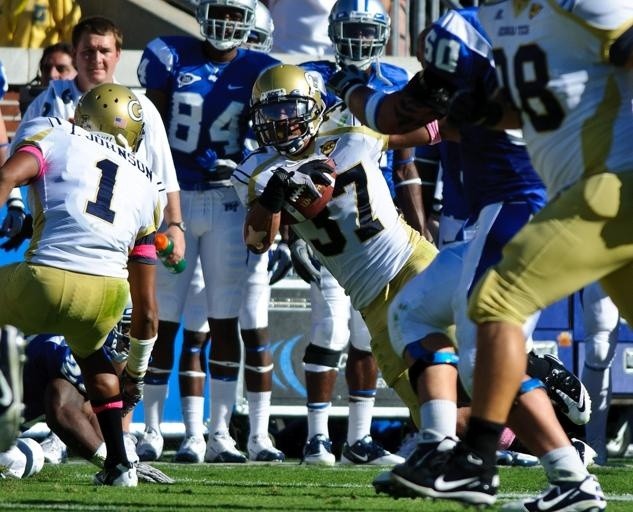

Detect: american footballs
[281, 158, 337, 225]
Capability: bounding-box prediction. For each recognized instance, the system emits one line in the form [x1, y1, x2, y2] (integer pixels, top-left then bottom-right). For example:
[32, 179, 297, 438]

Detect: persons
[0, 0, 633, 511]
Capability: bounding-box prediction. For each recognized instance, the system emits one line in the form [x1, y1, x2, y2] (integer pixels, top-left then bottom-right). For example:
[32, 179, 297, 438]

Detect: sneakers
[340, 433, 406, 467]
[173, 432, 207, 462]
[205, 431, 248, 463]
[40, 432, 69, 464]
[541, 354, 592, 425]
[499, 431, 607, 512]
[248, 437, 287, 461]
[372, 429, 500, 506]
[136, 433, 165, 461]
[94, 458, 138, 486]
[304, 433, 336, 467]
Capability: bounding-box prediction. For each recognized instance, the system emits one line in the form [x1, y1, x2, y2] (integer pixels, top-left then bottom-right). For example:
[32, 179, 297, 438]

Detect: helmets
[106, 300, 138, 363]
[194, 0, 273, 53]
[328, 0, 391, 71]
[72, 82, 145, 152]
[249, 62, 327, 156]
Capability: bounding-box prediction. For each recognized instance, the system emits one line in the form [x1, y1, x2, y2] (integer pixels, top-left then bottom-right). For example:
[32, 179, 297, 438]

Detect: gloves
[1, 199, 26, 238]
[259, 157, 333, 212]
[446, 89, 504, 133]
[325, 65, 369, 105]
[140, 461, 173, 485]
[288, 231, 324, 286]
[116, 370, 145, 417]
[268, 239, 291, 286]
